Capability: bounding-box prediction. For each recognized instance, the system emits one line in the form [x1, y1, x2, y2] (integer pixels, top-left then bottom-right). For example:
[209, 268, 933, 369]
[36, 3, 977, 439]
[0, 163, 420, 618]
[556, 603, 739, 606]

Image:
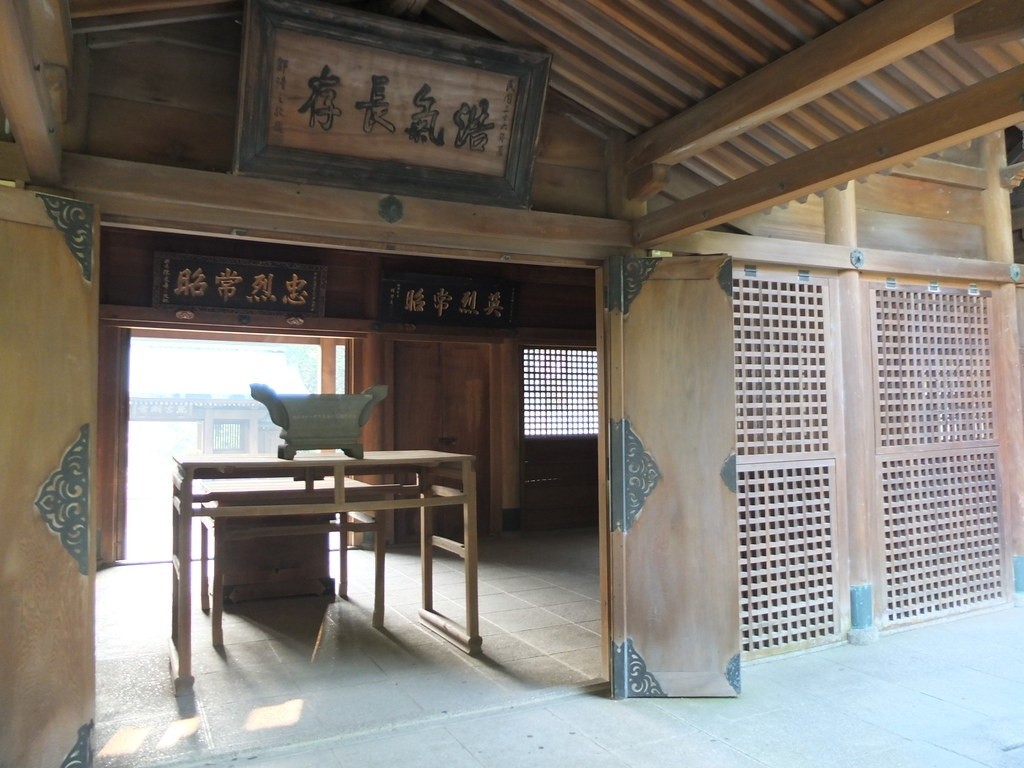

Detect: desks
[169, 450, 481, 686]
[199, 477, 387, 645]
